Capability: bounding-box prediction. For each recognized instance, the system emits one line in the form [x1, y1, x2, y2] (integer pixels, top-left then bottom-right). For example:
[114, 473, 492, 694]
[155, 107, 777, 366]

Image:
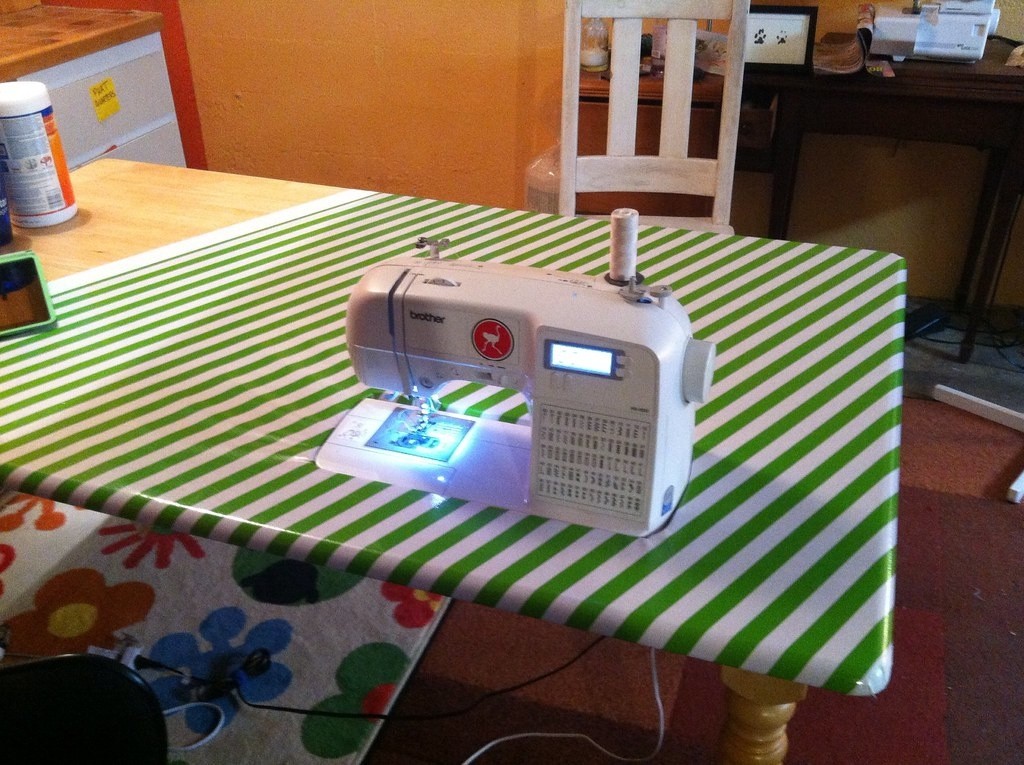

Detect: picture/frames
[744, 4, 819, 72]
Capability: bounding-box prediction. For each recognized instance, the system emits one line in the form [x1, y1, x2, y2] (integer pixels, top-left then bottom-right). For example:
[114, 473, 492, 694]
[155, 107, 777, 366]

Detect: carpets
[0, 486, 453, 765]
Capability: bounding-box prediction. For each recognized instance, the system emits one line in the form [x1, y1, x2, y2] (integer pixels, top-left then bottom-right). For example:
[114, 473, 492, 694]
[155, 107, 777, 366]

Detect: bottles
[649, 19, 667, 81]
[580, 18, 609, 72]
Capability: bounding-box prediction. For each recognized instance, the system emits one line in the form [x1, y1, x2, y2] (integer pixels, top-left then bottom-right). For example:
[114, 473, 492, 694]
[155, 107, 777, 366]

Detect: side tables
[576, 50, 724, 217]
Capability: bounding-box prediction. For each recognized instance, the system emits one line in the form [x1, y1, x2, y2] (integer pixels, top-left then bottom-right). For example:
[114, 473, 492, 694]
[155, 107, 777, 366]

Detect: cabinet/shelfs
[0, 0, 189, 176]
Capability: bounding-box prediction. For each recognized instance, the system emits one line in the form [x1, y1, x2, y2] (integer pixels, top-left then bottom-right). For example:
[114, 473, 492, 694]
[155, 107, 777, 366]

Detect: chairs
[558, 0, 751, 235]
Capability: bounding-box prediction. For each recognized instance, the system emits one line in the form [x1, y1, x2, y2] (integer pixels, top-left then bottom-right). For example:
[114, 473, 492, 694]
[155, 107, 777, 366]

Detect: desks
[743, 31, 1024, 364]
[0, 157, 909, 765]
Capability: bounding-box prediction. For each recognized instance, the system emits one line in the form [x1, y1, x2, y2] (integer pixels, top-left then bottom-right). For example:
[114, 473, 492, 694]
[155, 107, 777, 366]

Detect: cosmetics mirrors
[0, 249, 56, 337]
